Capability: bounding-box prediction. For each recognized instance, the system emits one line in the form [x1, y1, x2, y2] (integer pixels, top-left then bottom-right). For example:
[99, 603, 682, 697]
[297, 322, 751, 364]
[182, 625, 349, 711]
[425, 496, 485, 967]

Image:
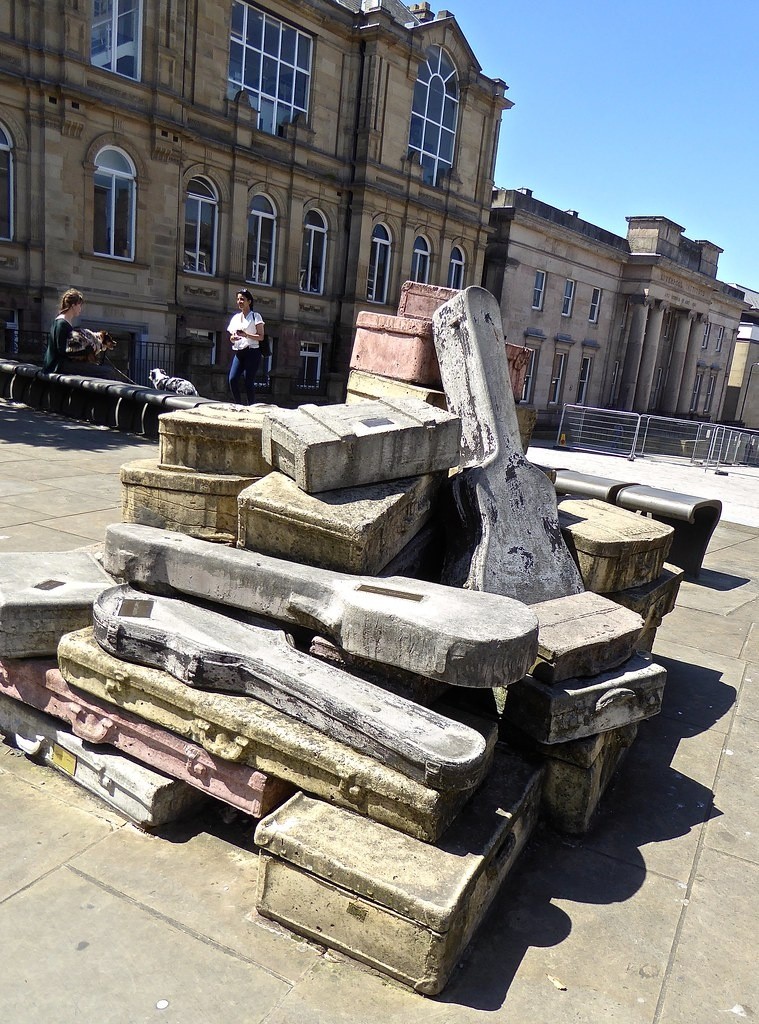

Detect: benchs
[0, 358, 220, 435]
[547, 468, 723, 583]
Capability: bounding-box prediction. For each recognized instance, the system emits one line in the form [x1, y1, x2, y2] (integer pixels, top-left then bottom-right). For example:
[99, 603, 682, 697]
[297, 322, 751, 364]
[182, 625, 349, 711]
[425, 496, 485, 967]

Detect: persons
[41, 288, 118, 381]
[226, 289, 266, 405]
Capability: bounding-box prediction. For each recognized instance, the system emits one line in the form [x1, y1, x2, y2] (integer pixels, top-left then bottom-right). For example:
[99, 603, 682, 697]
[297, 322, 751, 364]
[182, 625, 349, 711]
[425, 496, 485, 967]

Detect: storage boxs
[0, 281, 683, 996]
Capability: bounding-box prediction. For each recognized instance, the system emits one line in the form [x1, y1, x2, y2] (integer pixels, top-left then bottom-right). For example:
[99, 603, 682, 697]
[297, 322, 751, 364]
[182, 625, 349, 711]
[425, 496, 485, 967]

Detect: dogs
[65, 328, 117, 365]
[148, 368, 200, 397]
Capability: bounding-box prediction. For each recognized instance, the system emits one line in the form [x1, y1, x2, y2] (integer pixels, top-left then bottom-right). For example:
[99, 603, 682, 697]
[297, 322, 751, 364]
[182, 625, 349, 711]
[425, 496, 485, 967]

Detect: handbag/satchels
[253, 312, 272, 357]
